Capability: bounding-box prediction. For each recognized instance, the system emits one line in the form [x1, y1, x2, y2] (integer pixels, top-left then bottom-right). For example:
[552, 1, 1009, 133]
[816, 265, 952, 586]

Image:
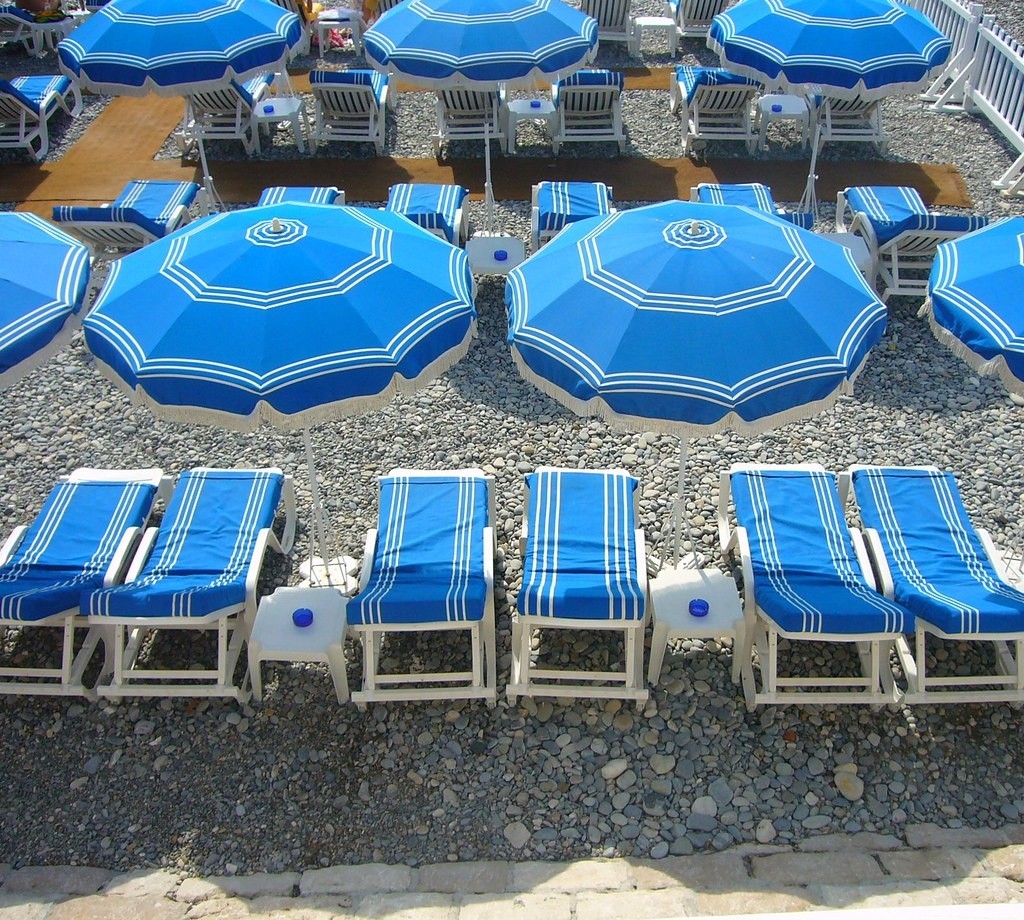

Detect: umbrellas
[706, 0, 953, 102]
[917, 217, 1024, 399]
[0, 211, 91, 395]
[82, 201, 479, 598]
[57, 0, 307, 212]
[502, 198, 887, 577]
[363, 0, 601, 238]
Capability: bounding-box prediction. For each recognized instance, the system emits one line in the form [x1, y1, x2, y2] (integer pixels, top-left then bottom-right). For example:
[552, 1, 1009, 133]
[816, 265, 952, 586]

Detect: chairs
[0, 6, 38, 57]
[344, 467, 499, 712]
[803, 93, 891, 156]
[308, 69, 394, 150]
[581, 0, 634, 55]
[430, 83, 507, 157]
[852, 458, 1024, 706]
[689, 183, 814, 232]
[550, 69, 626, 155]
[834, 186, 989, 304]
[530, 182, 618, 255]
[269, 0, 318, 56]
[257, 186, 346, 208]
[79, 466, 296, 708]
[663, 0, 730, 51]
[717, 463, 917, 716]
[0, 75, 84, 162]
[53, 178, 208, 267]
[669, 65, 762, 156]
[505, 466, 649, 712]
[174, 73, 275, 155]
[378, 183, 470, 248]
[0, 467, 175, 701]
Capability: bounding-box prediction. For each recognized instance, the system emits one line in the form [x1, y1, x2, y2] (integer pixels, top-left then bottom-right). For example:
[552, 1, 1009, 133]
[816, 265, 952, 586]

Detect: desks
[463, 237, 525, 274]
[30, 18, 74, 49]
[247, 586, 361, 706]
[633, 16, 675, 59]
[250, 97, 310, 154]
[318, 12, 362, 57]
[812, 232, 873, 291]
[753, 94, 810, 151]
[505, 99, 559, 154]
[648, 567, 746, 690]
